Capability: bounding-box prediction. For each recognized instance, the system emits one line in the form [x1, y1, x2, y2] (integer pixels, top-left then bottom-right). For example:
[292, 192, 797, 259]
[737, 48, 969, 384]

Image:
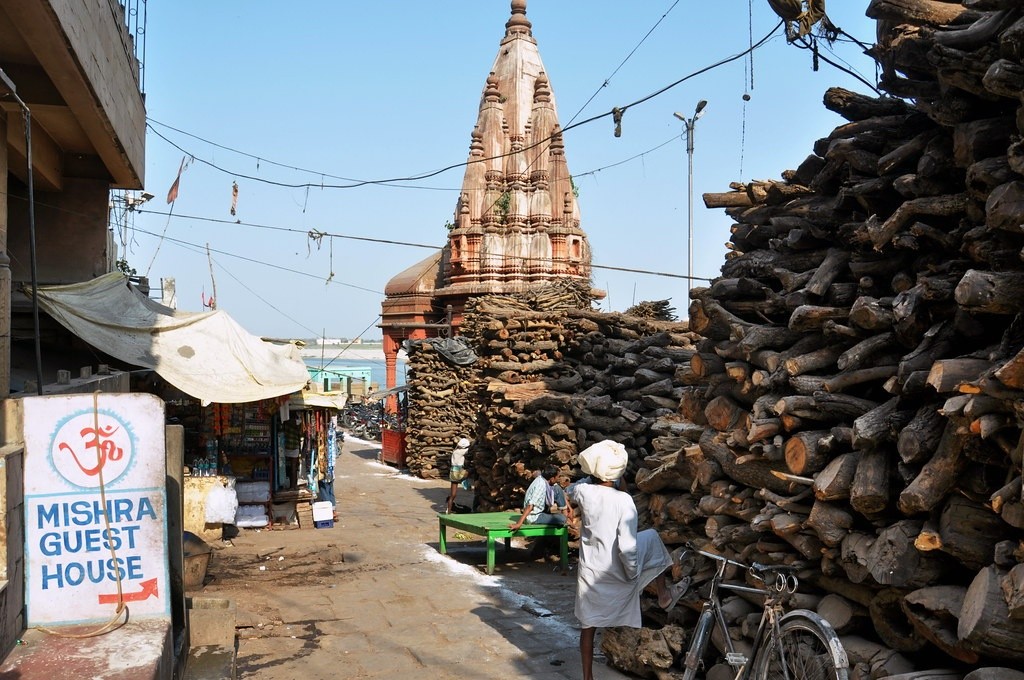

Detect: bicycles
[677, 548, 849, 680]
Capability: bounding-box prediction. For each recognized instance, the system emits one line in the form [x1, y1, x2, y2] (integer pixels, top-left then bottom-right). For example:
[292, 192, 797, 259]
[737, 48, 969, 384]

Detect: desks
[436, 511, 566, 575]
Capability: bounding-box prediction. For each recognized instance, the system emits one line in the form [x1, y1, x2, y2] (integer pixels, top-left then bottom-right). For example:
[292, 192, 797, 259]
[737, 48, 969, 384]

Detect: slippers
[665, 576, 692, 612]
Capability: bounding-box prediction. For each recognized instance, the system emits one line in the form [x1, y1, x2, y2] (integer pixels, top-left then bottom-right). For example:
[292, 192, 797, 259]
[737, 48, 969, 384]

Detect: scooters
[336, 397, 407, 441]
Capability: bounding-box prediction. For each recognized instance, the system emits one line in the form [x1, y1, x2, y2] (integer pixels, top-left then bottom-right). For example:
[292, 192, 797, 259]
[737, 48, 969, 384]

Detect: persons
[573, 440, 690, 680]
[368, 387, 374, 394]
[446, 438, 480, 514]
[314, 437, 339, 521]
[508, 464, 620, 539]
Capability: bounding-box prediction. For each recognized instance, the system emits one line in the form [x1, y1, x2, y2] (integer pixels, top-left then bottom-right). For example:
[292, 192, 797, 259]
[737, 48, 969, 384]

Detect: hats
[458, 438, 470, 448]
[577, 440, 627, 483]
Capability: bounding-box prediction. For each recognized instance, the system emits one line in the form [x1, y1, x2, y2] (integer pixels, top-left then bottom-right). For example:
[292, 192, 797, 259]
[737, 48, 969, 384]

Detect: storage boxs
[312, 500, 334, 529]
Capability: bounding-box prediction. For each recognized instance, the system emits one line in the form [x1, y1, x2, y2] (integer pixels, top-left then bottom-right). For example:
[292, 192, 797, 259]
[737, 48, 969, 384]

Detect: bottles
[193, 457, 198, 477]
[253, 465, 264, 477]
[204, 456, 211, 477]
[211, 455, 217, 477]
[198, 457, 204, 477]
